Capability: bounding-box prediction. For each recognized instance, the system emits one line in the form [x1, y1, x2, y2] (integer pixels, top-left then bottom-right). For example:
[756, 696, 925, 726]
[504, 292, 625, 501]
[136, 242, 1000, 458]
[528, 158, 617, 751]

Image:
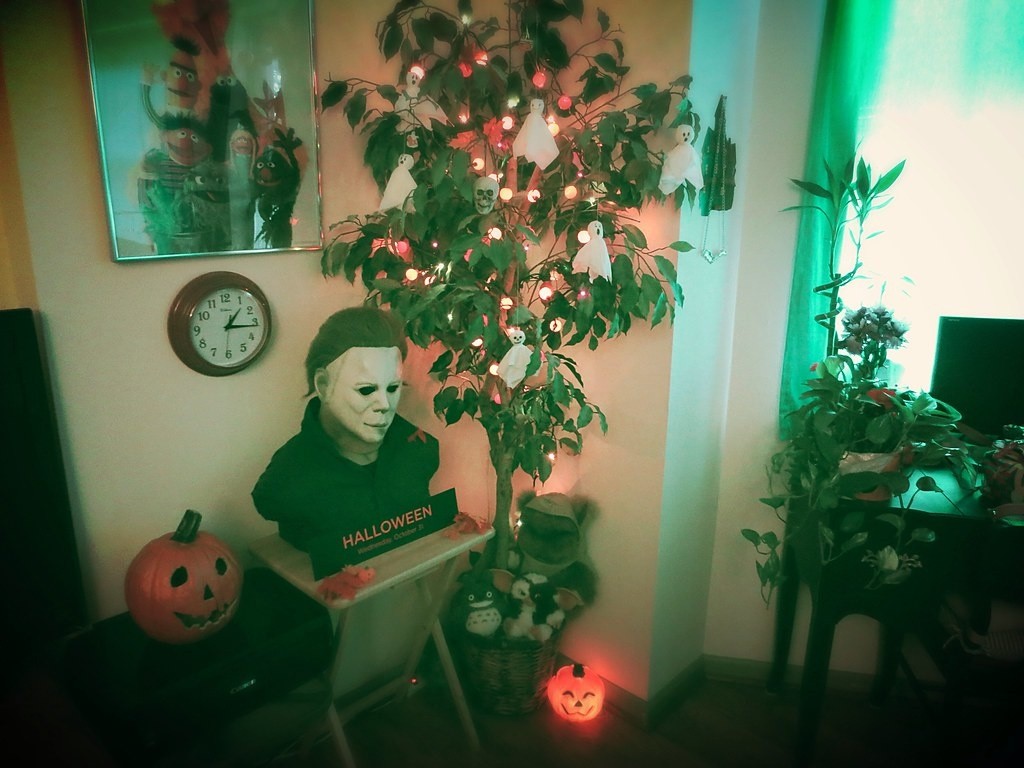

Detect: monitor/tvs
[930, 316, 1024, 435]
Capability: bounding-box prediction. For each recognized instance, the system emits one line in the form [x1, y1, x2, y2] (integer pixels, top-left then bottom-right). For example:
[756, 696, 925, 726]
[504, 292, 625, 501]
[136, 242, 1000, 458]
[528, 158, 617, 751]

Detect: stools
[876, 610, 1024, 768]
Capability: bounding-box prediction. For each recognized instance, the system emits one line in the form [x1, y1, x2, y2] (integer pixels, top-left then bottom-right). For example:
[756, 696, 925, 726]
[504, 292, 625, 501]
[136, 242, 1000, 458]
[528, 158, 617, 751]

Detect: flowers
[834, 303, 910, 379]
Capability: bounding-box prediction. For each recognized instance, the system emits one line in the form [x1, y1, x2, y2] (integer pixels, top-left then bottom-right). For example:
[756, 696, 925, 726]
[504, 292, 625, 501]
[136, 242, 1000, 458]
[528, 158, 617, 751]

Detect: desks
[764, 457, 1024, 768]
[247, 525, 496, 768]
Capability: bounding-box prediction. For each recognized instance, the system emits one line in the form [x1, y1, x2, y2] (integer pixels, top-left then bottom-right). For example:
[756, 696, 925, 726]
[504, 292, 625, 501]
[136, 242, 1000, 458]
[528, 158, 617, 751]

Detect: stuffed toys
[137, 34, 302, 248]
[454, 491, 596, 641]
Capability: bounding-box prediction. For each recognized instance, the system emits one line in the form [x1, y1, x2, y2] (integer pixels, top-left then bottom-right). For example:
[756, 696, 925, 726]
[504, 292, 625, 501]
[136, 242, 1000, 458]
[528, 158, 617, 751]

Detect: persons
[251, 306, 439, 552]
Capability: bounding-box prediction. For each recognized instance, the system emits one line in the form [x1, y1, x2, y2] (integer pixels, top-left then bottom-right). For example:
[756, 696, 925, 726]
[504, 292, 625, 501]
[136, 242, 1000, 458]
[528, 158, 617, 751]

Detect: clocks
[167, 271, 272, 377]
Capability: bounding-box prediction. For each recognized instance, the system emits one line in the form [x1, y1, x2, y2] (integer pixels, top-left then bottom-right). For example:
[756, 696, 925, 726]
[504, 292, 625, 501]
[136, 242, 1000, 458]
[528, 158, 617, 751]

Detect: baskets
[453, 620, 567, 717]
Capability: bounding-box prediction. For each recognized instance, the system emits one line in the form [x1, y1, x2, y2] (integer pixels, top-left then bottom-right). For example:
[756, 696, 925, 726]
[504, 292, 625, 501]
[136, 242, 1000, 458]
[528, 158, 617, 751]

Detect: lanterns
[125, 510, 244, 643]
[548, 663, 605, 723]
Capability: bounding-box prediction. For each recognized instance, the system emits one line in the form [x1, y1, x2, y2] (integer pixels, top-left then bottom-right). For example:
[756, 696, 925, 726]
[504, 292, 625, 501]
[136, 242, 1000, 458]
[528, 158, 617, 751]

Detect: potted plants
[742, 354, 1019, 610]
[320, 0, 702, 717]
